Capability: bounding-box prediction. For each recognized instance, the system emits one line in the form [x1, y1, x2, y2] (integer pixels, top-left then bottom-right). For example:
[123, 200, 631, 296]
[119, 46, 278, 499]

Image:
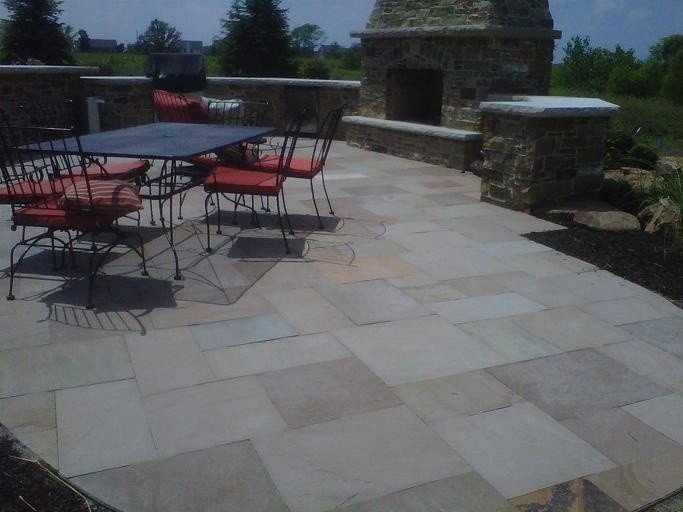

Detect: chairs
[0, 126, 148, 309]
[172, 100, 267, 176]
[203, 106, 308, 255]
[251, 104, 346, 231]
[150, 89, 195, 124]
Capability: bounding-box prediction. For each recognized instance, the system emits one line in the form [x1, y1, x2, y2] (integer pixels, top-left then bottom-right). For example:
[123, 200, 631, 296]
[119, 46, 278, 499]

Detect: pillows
[59, 178, 144, 213]
[202, 96, 245, 125]
[153, 92, 203, 121]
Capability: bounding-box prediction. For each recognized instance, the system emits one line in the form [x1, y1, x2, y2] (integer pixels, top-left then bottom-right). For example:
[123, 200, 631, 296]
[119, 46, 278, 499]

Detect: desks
[11, 120, 281, 280]
